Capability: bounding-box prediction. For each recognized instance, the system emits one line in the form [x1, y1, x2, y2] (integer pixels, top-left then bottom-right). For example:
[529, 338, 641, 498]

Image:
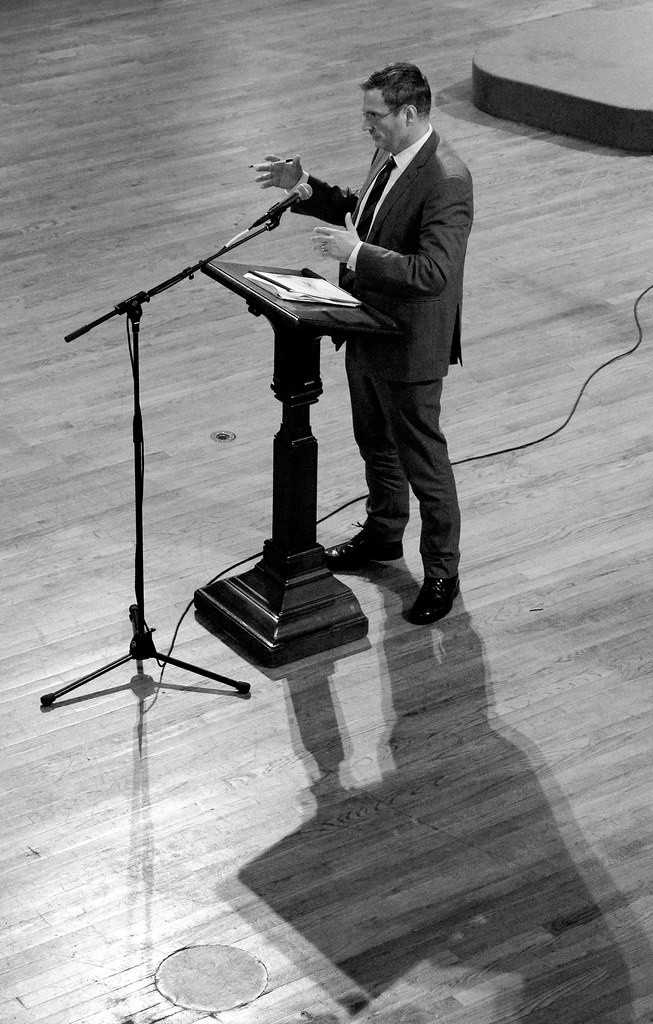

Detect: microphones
[252, 183, 313, 227]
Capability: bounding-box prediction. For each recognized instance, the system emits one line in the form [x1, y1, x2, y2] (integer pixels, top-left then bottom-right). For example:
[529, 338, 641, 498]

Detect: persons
[254, 62, 476, 625]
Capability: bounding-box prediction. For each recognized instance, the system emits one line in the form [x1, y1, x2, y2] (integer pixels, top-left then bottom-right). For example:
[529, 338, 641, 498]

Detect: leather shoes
[410, 574, 460, 624]
[324, 523, 403, 569]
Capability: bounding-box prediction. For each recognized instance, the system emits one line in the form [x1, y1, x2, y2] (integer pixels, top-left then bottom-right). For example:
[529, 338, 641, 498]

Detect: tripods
[37, 214, 281, 707]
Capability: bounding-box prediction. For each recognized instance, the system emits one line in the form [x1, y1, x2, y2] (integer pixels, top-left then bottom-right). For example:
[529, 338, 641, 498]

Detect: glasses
[359, 105, 409, 125]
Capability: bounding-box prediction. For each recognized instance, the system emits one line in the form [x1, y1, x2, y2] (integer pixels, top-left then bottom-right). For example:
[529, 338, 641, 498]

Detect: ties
[356, 157, 397, 242]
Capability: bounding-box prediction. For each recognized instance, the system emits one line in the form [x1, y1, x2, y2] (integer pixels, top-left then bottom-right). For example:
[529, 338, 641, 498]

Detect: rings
[322, 244, 328, 252]
